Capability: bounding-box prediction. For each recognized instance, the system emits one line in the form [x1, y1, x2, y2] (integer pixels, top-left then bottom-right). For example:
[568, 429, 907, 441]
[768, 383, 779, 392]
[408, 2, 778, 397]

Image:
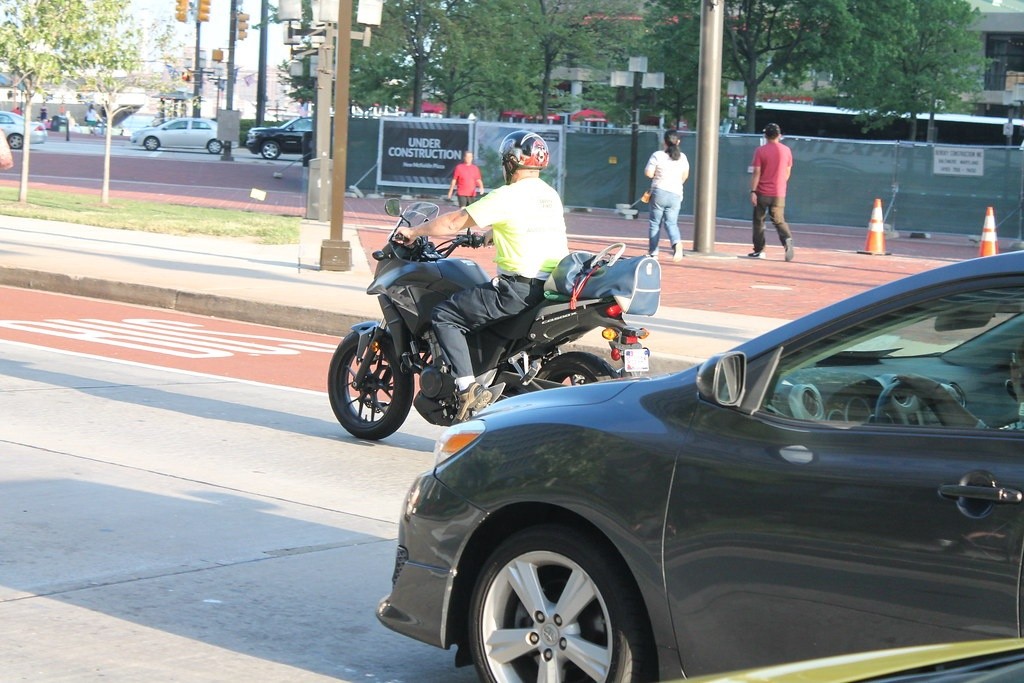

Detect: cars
[376, 252, 1024, 683]
[0, 110, 48, 149]
[130, 117, 240, 154]
[59, 116, 78, 126]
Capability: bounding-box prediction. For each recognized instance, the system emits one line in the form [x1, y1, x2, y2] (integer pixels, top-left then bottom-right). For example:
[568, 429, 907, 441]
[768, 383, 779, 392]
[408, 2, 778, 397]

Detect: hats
[762, 123, 779, 132]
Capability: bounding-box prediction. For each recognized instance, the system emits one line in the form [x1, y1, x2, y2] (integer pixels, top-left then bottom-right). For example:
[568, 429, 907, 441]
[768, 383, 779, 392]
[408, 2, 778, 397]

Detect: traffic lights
[199, 0, 210, 22]
[182, 74, 190, 81]
[238, 14, 249, 41]
[176, 0, 188, 23]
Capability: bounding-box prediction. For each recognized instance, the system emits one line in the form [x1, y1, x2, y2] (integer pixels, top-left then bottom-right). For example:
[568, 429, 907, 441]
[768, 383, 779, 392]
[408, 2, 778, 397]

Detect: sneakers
[451, 381, 492, 426]
[748, 252, 764, 258]
[784, 238, 793, 261]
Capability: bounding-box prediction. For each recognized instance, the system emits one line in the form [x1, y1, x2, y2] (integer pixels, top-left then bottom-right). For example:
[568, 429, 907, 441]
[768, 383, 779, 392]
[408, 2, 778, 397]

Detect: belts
[501, 274, 545, 286]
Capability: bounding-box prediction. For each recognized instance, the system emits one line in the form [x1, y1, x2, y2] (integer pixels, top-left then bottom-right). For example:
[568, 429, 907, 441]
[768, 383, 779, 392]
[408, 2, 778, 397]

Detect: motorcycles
[327, 198, 651, 442]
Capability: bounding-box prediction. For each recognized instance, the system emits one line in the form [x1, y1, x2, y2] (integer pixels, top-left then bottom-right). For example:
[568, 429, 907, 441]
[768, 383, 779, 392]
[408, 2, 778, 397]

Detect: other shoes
[673, 242, 683, 261]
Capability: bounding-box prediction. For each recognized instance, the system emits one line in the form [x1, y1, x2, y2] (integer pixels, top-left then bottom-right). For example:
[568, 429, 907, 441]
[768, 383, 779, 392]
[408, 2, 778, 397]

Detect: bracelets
[751, 191, 756, 193]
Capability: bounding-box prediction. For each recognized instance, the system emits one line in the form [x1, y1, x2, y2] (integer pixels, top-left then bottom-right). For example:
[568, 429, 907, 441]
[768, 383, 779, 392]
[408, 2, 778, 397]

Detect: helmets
[499, 130, 549, 169]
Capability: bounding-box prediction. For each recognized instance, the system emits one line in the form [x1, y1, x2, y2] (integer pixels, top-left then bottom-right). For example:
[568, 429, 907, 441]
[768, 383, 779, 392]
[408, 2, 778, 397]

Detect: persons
[893, 341, 1024, 430]
[747, 122, 794, 261]
[40, 102, 47, 120]
[645, 130, 690, 262]
[392, 130, 570, 425]
[448, 151, 485, 208]
[87, 104, 97, 126]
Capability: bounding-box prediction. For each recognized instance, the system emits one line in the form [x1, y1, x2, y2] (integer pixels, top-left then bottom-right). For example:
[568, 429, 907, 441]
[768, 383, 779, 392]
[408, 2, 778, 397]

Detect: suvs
[246, 116, 313, 159]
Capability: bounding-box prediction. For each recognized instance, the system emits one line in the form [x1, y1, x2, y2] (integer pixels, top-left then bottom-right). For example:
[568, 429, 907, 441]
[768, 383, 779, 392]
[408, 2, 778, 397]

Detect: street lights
[611, 56, 665, 206]
[1001, 84, 1024, 211]
[277, 0, 383, 221]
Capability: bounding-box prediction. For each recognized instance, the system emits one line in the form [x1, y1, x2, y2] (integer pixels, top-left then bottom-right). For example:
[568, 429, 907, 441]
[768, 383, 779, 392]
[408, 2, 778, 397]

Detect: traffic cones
[857, 198, 892, 255]
[978, 207, 1000, 258]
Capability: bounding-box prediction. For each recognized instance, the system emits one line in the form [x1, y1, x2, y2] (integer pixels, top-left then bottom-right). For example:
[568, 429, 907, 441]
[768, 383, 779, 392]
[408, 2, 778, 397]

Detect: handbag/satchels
[641, 190, 651, 203]
[543, 243, 661, 315]
